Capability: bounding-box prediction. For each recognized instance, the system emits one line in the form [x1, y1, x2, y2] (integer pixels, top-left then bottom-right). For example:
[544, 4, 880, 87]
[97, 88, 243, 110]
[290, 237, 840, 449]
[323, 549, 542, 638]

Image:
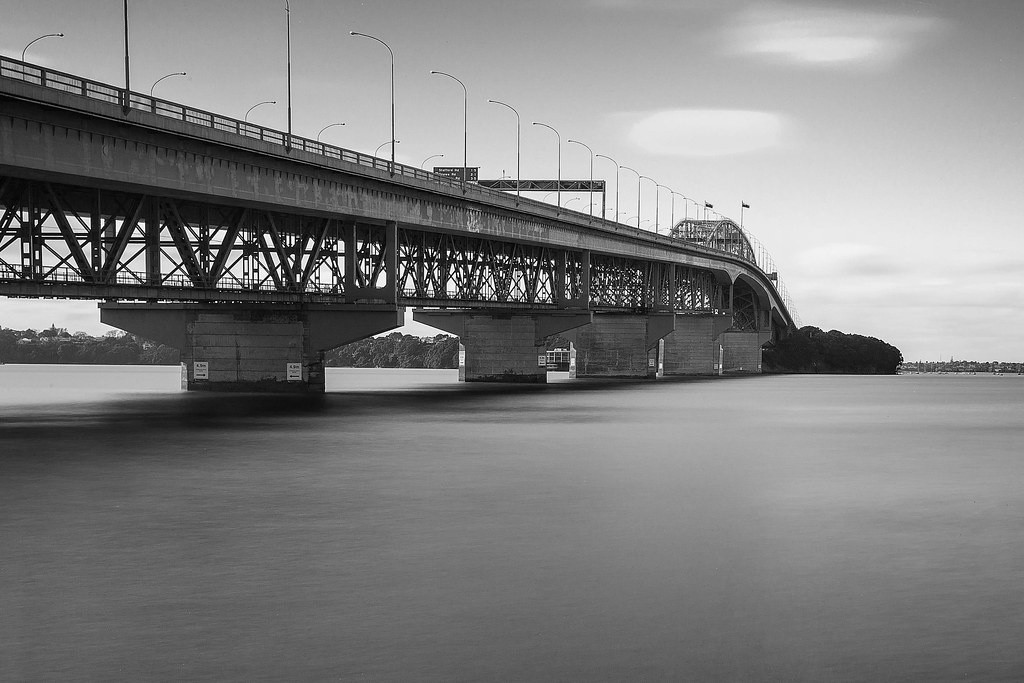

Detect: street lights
[429, 69, 467, 195]
[244, 101, 276, 136]
[625, 207, 800, 329]
[598, 209, 613, 218]
[151, 72, 187, 98]
[317, 123, 346, 154]
[488, 100, 520, 209]
[595, 155, 619, 231]
[568, 140, 593, 225]
[671, 191, 688, 248]
[350, 31, 395, 172]
[583, 204, 598, 213]
[639, 175, 658, 240]
[657, 184, 673, 245]
[532, 122, 561, 219]
[421, 155, 444, 179]
[694, 203, 709, 251]
[612, 213, 627, 222]
[620, 166, 641, 236]
[684, 197, 698, 250]
[21, 32, 64, 81]
[564, 198, 581, 208]
[374, 140, 400, 157]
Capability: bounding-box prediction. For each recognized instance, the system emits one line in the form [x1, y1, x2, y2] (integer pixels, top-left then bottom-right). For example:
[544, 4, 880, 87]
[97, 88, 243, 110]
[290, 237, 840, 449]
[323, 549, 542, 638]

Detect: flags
[742, 202, 749, 208]
[704, 202, 713, 208]
